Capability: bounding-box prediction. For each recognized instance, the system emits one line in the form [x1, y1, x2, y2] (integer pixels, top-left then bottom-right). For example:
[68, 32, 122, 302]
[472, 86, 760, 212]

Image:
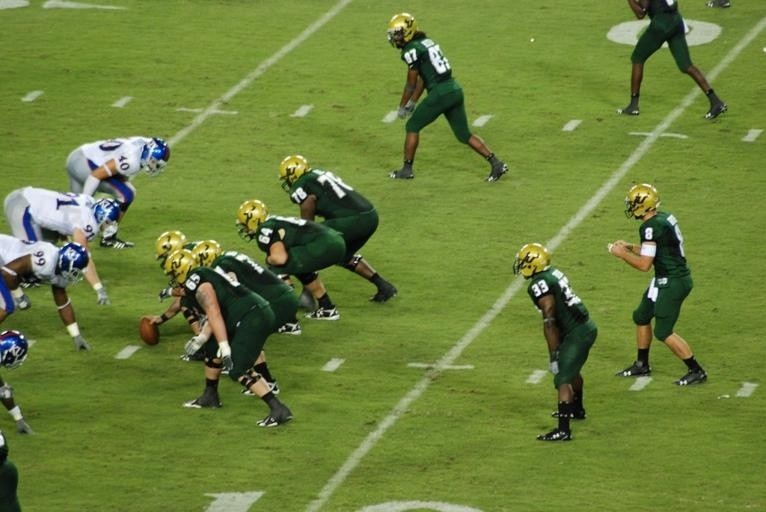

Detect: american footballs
[140, 317, 159, 344]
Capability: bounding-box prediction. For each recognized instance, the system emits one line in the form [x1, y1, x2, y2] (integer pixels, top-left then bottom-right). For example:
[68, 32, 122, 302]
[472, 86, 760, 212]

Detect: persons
[622, 0, 727, 120]
[140, 230, 298, 427]
[606, 184, 707, 386]
[278, 154, 397, 310]
[388, 13, 508, 183]
[512, 243, 596, 441]
[3, 186, 119, 309]
[0, 234, 91, 351]
[67, 137, 170, 248]
[235, 199, 346, 335]
[0, 330, 33, 433]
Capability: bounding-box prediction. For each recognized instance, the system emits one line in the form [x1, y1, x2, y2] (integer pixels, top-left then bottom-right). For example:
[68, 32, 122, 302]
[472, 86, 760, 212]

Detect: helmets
[234, 199, 270, 243]
[623, 183, 663, 220]
[0, 329, 32, 370]
[512, 242, 553, 280]
[385, 12, 418, 49]
[56, 241, 90, 285]
[278, 154, 310, 187]
[140, 137, 170, 176]
[153, 229, 223, 285]
[91, 197, 121, 231]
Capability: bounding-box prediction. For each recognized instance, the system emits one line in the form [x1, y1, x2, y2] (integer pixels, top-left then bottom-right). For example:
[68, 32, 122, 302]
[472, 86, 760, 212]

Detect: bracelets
[66, 322, 80, 337]
[92, 283, 102, 290]
[11, 286, 24, 299]
[8, 405, 23, 422]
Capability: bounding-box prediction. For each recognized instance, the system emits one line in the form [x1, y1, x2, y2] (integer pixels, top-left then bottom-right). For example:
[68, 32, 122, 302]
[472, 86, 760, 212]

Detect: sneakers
[705, 1, 731, 8]
[614, 361, 652, 379]
[303, 306, 341, 321]
[699, 101, 729, 120]
[368, 284, 398, 303]
[386, 165, 415, 179]
[484, 160, 509, 184]
[551, 407, 586, 421]
[99, 231, 135, 249]
[182, 397, 223, 409]
[256, 414, 295, 427]
[615, 104, 643, 120]
[240, 381, 280, 396]
[277, 321, 302, 335]
[672, 367, 708, 387]
[537, 427, 573, 441]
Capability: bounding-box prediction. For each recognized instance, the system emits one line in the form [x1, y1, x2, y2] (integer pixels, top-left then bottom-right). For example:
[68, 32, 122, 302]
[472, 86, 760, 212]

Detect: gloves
[397, 98, 415, 119]
[184, 333, 209, 356]
[215, 340, 234, 375]
[14, 417, 35, 436]
[12, 293, 32, 311]
[95, 287, 112, 306]
[157, 285, 173, 303]
[70, 334, 92, 352]
[548, 349, 560, 377]
